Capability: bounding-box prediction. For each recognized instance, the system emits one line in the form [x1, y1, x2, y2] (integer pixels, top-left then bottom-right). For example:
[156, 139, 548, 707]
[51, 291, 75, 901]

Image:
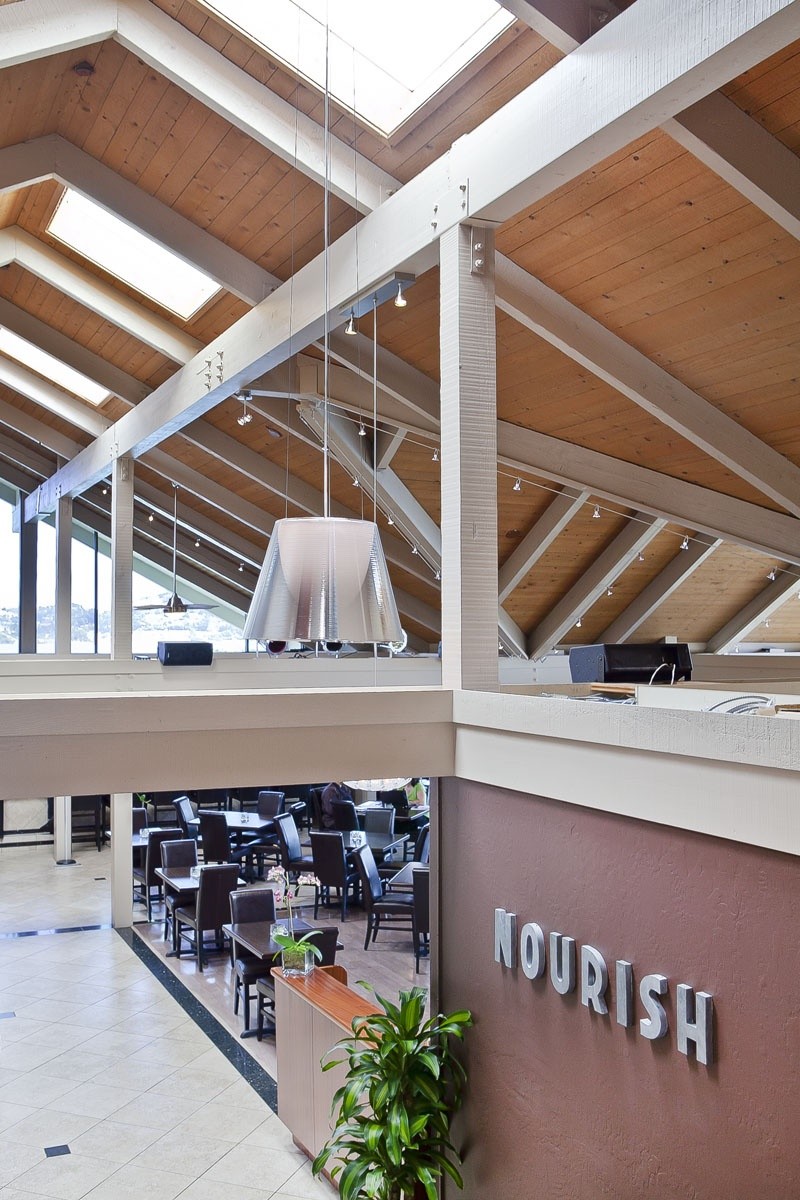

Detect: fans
[130, 489, 218, 615]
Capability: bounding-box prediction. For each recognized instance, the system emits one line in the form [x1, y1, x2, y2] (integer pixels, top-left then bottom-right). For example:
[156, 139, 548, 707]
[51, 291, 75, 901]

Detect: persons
[319, 781, 355, 829]
[397, 777, 427, 806]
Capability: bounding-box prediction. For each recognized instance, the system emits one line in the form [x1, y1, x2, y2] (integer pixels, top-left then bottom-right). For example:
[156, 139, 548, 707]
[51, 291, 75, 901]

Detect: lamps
[241, 16, 406, 653]
[336, 269, 415, 337]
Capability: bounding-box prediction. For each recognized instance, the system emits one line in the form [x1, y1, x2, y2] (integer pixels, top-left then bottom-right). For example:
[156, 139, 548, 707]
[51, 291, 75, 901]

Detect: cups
[190, 866, 200, 878]
[241, 812, 249, 820]
[139, 828, 150, 838]
[351, 830, 363, 842]
[269, 923, 288, 936]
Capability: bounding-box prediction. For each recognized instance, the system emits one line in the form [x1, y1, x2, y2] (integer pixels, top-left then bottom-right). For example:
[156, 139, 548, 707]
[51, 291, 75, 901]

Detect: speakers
[567, 643, 692, 684]
[156, 640, 212, 666]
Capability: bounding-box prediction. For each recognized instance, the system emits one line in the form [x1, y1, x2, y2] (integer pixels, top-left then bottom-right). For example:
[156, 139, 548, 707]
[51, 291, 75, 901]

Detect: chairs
[73, 778, 431, 1042]
